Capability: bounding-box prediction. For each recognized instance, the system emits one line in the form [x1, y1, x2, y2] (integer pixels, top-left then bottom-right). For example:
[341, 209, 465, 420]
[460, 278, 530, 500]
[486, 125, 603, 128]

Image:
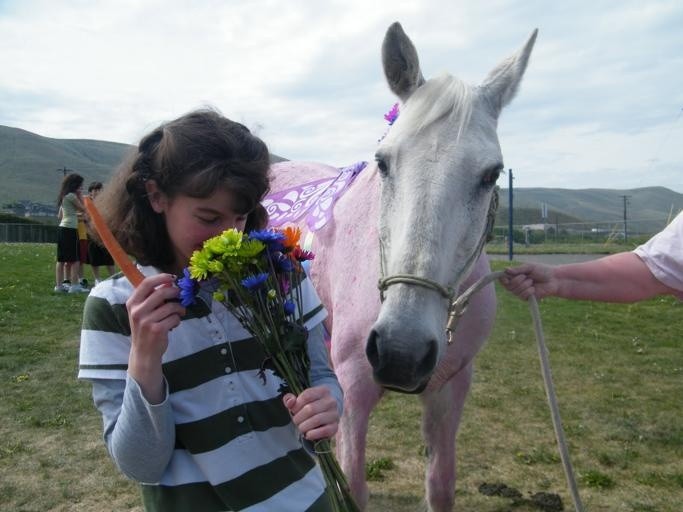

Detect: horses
[84, 22, 538, 511]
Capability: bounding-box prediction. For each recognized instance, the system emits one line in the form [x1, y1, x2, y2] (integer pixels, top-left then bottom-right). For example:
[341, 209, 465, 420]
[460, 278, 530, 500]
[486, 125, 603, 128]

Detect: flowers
[179, 226, 364, 511]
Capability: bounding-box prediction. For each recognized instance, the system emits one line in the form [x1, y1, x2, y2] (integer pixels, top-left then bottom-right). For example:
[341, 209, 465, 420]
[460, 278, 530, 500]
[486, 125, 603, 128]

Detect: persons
[83, 180, 124, 288]
[50, 174, 81, 294]
[498, 206, 682, 304]
[72, 196, 93, 294]
[76, 103, 358, 510]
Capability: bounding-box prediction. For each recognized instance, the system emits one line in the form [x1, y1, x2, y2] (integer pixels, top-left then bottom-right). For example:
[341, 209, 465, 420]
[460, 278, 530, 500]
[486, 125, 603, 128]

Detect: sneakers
[54, 278, 90, 294]
